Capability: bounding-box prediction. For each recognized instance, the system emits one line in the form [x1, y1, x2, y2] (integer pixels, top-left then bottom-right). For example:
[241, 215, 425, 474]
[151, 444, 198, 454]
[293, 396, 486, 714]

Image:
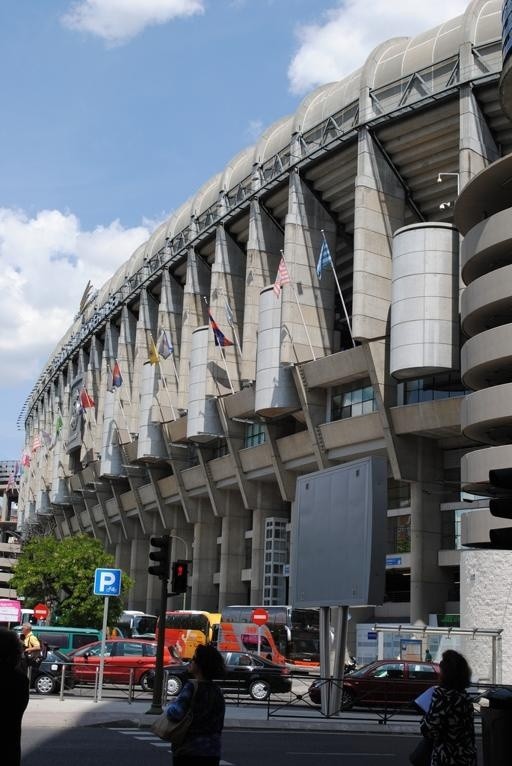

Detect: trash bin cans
[473, 687, 512, 766]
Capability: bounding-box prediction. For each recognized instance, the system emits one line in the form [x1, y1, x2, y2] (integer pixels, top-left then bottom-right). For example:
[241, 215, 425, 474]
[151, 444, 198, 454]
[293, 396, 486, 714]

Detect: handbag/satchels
[408, 735, 434, 766]
[149, 698, 196, 745]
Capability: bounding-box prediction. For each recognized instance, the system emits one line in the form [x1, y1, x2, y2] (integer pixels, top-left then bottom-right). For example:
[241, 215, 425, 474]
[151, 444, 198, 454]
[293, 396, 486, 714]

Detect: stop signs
[34, 604, 49, 620]
[250, 608, 268, 627]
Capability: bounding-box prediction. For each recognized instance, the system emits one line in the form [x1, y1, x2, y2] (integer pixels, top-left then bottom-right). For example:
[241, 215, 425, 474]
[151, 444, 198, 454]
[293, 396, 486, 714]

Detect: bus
[219, 603, 334, 675]
[155, 610, 222, 661]
[0, 609, 39, 632]
[117, 611, 156, 640]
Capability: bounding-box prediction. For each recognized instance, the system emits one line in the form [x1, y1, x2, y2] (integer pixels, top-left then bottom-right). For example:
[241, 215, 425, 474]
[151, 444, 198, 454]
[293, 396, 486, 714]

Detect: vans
[11, 626, 103, 653]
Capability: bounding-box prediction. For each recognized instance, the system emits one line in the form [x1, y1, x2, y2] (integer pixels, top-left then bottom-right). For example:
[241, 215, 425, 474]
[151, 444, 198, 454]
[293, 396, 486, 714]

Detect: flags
[272, 255, 291, 297]
[5, 359, 123, 495]
[206, 303, 235, 346]
[157, 330, 174, 359]
[149, 338, 160, 365]
[223, 296, 235, 327]
[314, 240, 331, 280]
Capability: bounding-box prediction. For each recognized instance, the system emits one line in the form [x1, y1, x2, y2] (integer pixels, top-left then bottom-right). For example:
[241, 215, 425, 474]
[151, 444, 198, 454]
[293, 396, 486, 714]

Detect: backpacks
[24, 645, 47, 666]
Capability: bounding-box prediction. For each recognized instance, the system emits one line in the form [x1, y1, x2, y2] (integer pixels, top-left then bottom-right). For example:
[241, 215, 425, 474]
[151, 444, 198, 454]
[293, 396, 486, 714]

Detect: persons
[425, 648, 432, 662]
[416, 649, 479, 765]
[166, 643, 226, 765]
[21, 621, 40, 652]
[0, 628, 32, 766]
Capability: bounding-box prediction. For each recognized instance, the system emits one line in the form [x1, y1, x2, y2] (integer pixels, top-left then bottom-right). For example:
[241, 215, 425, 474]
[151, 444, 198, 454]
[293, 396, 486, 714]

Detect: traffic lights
[147, 534, 171, 580]
[173, 562, 187, 593]
[488, 466, 512, 549]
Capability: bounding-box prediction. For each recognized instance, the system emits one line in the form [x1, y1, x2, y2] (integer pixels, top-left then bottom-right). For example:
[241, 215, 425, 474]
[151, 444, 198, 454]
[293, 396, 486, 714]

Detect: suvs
[67, 639, 171, 692]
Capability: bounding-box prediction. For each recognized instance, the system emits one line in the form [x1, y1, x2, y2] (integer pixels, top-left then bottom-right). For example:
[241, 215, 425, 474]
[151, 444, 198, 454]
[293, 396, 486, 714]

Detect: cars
[149, 649, 292, 701]
[310, 659, 481, 719]
[29, 651, 75, 696]
